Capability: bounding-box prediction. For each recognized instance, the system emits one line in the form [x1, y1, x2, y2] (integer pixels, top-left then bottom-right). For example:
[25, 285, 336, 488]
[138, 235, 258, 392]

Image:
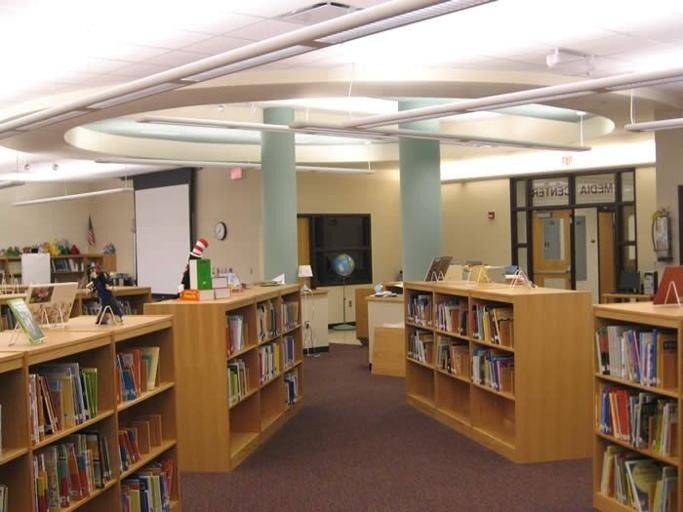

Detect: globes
[332, 253, 357, 331]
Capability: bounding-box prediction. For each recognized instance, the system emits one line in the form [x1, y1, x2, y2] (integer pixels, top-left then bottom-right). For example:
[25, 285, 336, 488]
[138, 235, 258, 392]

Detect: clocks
[213, 222, 227, 240]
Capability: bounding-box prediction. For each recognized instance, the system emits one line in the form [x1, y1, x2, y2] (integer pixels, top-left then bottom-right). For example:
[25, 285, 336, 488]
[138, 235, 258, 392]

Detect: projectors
[0, 180, 26, 188]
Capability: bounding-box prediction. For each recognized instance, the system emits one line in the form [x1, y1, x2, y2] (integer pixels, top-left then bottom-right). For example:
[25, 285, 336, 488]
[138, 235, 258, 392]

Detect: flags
[87, 215, 97, 246]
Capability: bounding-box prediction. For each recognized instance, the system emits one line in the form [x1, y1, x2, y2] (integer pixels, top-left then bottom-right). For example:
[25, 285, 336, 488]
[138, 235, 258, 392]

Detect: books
[590, 321, 680, 511]
[180, 258, 300, 406]
[1, 238, 177, 512]
[400, 295, 535, 397]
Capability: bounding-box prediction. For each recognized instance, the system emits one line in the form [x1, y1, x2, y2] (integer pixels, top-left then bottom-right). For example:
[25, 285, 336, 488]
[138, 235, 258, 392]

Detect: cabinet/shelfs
[2, 250, 300, 510]
[590, 283, 682, 512]
[355, 267, 600, 467]
[301, 288, 330, 353]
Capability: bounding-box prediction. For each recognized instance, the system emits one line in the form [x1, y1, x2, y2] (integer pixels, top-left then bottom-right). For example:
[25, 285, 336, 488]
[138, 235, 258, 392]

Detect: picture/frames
[655, 215, 673, 262]
